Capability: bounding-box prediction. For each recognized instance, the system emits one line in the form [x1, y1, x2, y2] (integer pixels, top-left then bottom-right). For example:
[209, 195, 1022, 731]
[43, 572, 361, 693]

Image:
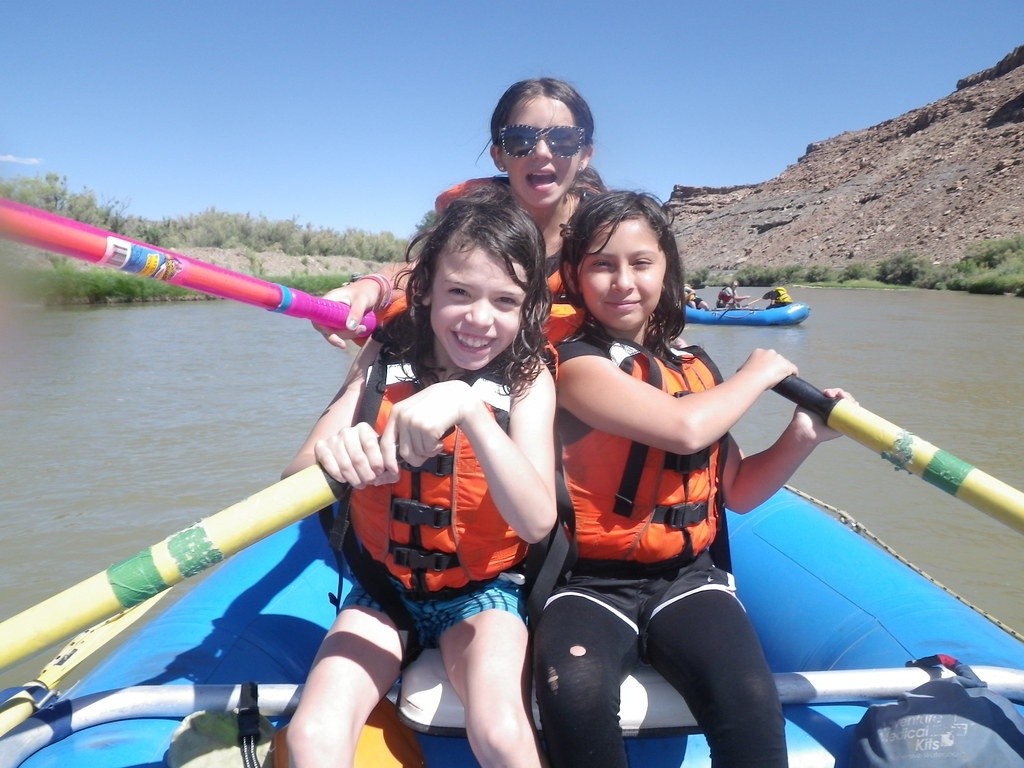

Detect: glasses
[500, 124, 585, 159]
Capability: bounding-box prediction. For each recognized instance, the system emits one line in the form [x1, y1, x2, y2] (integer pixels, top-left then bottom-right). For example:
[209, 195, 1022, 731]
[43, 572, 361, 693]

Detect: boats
[15, 462, 1024, 768]
[683, 303, 811, 326]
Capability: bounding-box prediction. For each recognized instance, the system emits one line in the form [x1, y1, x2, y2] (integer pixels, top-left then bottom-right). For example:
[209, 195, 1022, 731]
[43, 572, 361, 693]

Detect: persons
[683, 285, 710, 310]
[311, 78, 607, 358]
[531, 192, 860, 768]
[764, 286, 792, 309]
[272, 189, 556, 768]
[715, 280, 749, 307]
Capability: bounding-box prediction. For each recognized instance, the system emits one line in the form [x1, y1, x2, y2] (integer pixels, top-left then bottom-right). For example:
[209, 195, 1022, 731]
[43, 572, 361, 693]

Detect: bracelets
[351, 271, 392, 312]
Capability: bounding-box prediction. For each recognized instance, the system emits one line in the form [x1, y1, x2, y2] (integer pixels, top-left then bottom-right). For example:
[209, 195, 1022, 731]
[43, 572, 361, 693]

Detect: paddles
[742, 297, 763, 307]
[0, 584, 178, 741]
[769, 372, 1024, 537]
[0, 421, 459, 677]
